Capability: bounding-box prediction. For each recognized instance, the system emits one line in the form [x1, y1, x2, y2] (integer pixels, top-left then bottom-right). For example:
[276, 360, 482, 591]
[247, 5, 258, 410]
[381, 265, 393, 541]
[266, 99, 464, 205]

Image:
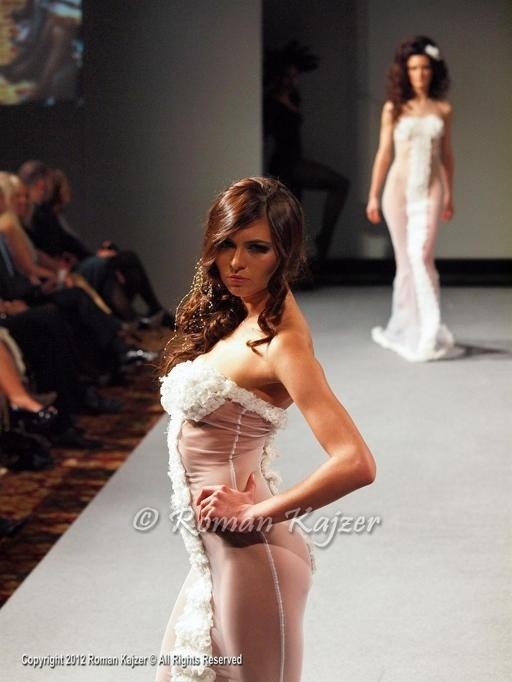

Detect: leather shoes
[50, 428, 102, 451]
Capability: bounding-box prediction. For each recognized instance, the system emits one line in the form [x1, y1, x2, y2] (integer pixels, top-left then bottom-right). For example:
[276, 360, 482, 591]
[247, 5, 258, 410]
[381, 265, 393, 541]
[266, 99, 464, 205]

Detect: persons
[157, 174, 380, 680]
[365, 34, 458, 367]
[1, 157, 176, 484]
[0, 0, 84, 107]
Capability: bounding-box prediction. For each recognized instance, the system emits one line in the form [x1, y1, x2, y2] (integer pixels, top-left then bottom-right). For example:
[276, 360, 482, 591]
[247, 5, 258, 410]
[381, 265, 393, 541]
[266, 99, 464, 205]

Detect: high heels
[8, 402, 58, 428]
